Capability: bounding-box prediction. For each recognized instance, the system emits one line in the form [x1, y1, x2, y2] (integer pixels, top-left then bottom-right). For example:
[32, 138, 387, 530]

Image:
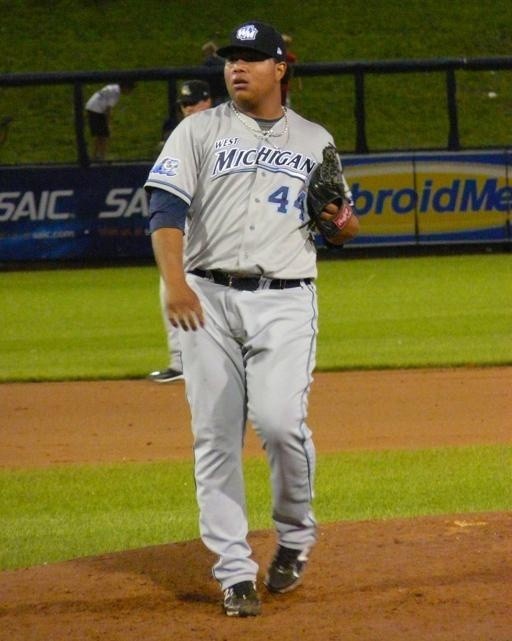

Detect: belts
[190, 269, 311, 291]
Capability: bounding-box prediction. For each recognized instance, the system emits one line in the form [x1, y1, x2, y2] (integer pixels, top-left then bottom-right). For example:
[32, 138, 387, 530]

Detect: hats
[177, 81, 210, 104]
[216, 23, 287, 61]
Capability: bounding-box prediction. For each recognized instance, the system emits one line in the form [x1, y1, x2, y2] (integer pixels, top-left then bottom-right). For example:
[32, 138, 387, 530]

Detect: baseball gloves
[307, 144, 351, 250]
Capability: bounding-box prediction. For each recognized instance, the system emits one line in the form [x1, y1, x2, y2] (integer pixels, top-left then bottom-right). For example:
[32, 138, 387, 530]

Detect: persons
[143, 21, 360, 618]
[85, 78, 137, 160]
[175, 32, 321, 152]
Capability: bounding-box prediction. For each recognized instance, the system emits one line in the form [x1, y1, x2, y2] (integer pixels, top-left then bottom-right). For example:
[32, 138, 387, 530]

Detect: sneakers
[223, 581, 261, 615]
[265, 547, 305, 592]
[149, 369, 184, 383]
